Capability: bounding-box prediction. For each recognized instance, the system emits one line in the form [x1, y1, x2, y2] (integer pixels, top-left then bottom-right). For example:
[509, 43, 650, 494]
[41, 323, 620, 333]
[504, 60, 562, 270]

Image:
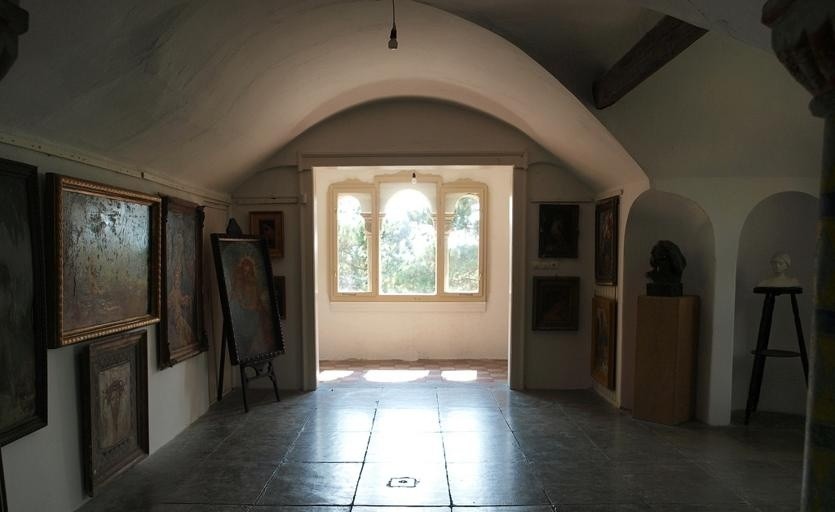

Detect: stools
[745, 287, 809, 420]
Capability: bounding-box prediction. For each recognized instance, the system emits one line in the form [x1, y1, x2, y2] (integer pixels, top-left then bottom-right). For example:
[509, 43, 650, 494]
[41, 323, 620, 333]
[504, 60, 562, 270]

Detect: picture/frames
[532, 198, 619, 391]
[157, 192, 209, 370]
[247, 210, 283, 259]
[44, 171, 160, 351]
[77, 328, 151, 499]
[211, 232, 283, 365]
[1, 159, 47, 449]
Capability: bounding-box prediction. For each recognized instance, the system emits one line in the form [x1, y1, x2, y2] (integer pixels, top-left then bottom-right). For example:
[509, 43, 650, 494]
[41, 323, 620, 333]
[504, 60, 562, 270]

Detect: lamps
[387, 1, 399, 50]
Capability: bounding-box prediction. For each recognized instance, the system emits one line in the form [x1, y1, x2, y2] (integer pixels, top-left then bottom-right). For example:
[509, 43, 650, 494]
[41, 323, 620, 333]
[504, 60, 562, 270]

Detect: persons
[754, 250, 804, 288]
[228, 257, 277, 355]
